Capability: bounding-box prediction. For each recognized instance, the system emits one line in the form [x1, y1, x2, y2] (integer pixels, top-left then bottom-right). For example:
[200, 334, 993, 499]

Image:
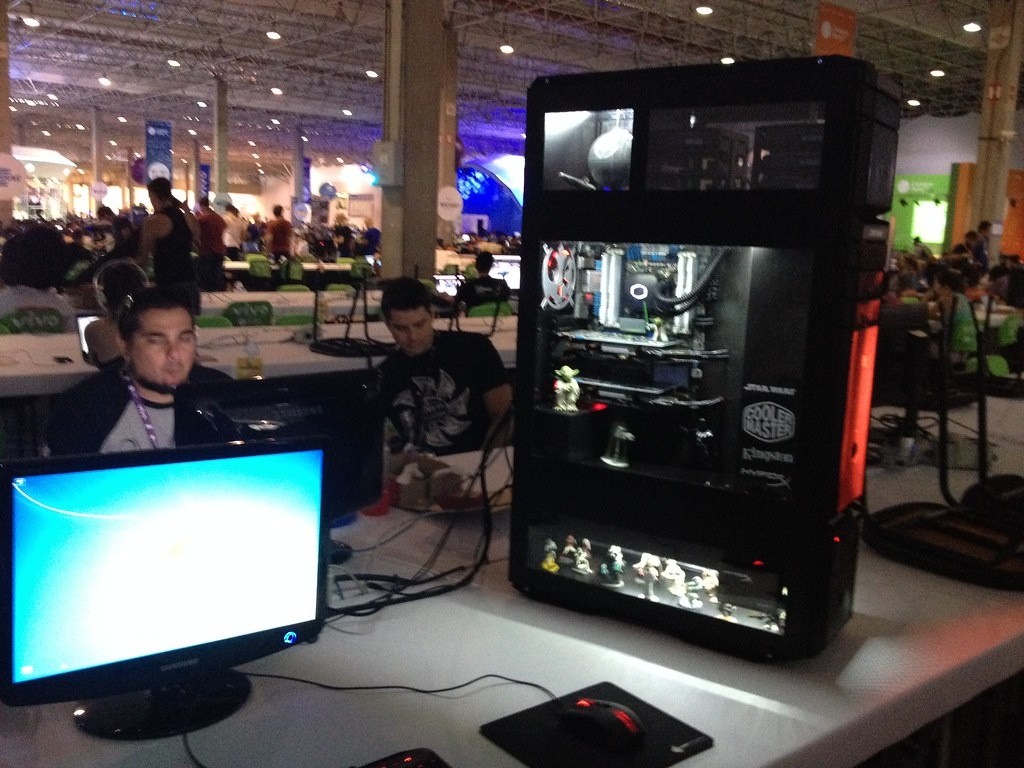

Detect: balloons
[132, 159, 148, 185]
[320, 183, 335, 196]
[295, 203, 311, 221]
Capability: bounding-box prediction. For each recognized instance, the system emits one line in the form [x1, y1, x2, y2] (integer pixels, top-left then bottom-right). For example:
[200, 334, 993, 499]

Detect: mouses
[554, 696, 646, 745]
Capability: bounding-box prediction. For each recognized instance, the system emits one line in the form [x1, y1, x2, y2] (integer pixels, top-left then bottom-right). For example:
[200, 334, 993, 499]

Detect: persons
[351, 217, 381, 256]
[265, 206, 292, 284]
[86, 265, 143, 370]
[131, 178, 202, 315]
[96, 206, 136, 258]
[370, 280, 515, 473]
[197, 197, 227, 292]
[222, 205, 252, 262]
[443, 251, 512, 304]
[334, 214, 350, 258]
[453, 227, 522, 254]
[249, 213, 266, 241]
[35, 291, 261, 456]
[884, 221, 1024, 381]
[59, 231, 94, 294]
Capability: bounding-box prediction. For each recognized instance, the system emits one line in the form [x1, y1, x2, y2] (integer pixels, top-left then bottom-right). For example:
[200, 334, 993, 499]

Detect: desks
[0, 316, 520, 400]
[200, 289, 382, 319]
[0, 392, 1024, 767]
[222, 260, 354, 272]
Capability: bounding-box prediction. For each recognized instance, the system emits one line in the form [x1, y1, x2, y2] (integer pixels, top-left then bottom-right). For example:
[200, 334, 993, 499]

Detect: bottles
[359, 440, 391, 516]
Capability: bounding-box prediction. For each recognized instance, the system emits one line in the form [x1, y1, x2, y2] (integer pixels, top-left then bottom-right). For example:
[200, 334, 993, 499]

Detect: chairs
[0, 252, 513, 333]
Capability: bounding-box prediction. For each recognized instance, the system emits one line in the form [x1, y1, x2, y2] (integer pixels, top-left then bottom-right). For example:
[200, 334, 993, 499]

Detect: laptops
[74, 314, 104, 362]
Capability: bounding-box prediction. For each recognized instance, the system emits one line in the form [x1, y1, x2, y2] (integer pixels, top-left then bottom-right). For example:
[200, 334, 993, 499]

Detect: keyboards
[358, 748, 453, 768]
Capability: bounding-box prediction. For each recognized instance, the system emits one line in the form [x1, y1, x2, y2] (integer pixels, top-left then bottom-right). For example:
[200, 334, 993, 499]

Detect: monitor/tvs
[0, 363, 387, 742]
